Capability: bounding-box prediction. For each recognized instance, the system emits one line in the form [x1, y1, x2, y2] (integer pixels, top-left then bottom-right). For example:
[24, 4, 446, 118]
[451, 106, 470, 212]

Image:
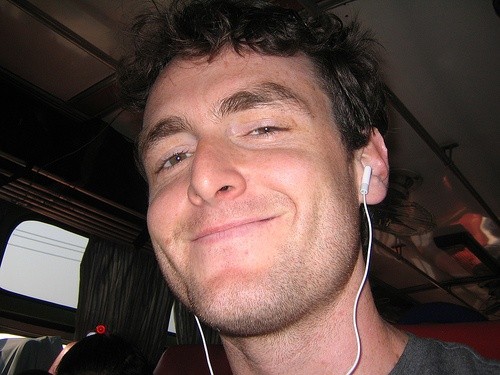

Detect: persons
[111, 0, 500, 375]
[56, 335, 153, 375]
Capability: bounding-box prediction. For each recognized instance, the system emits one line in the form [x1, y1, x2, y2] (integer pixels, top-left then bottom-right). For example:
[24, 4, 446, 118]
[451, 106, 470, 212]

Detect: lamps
[434, 232, 497, 276]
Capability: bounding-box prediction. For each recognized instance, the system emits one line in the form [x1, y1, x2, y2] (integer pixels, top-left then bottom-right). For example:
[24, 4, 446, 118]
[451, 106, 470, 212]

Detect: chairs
[154, 320, 499, 375]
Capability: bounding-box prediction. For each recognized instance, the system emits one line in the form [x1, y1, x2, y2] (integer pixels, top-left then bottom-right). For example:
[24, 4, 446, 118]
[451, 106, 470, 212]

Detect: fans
[356, 170, 435, 236]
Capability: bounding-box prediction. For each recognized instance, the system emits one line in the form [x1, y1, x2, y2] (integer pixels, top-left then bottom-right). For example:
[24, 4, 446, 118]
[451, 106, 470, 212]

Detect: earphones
[359, 166, 371, 194]
